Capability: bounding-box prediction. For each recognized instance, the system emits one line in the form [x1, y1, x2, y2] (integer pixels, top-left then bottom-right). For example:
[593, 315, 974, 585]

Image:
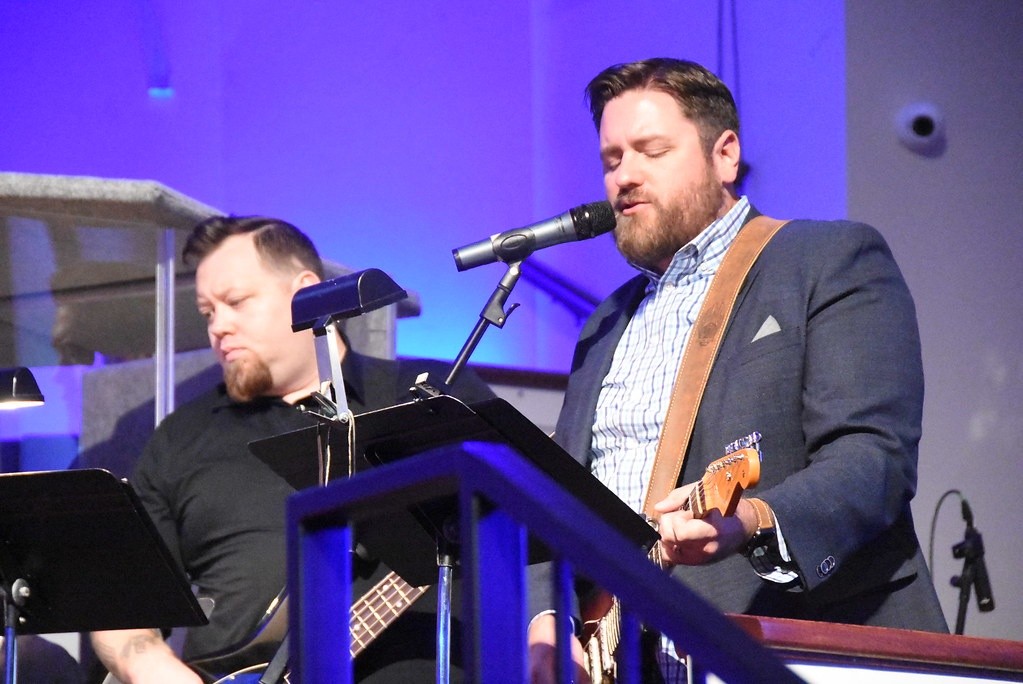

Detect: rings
[672, 543, 681, 554]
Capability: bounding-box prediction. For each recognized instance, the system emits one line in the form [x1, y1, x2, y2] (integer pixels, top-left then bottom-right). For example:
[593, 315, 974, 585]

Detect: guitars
[577, 429, 764, 684]
[100, 431, 557, 684]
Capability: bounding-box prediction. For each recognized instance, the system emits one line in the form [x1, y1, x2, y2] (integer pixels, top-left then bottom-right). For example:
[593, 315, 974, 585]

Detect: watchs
[741, 496, 776, 558]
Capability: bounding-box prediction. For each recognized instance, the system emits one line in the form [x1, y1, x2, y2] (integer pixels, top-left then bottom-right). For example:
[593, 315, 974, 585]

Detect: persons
[0, 634, 79, 684]
[527, 57, 952, 684]
[88, 214, 503, 684]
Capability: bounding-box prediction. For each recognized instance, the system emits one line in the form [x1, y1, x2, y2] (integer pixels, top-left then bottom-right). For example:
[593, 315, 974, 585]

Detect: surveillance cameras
[896, 103, 944, 151]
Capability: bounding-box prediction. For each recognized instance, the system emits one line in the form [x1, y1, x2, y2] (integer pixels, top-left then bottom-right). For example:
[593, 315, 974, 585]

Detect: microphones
[961, 499, 996, 612]
[451, 200, 617, 272]
